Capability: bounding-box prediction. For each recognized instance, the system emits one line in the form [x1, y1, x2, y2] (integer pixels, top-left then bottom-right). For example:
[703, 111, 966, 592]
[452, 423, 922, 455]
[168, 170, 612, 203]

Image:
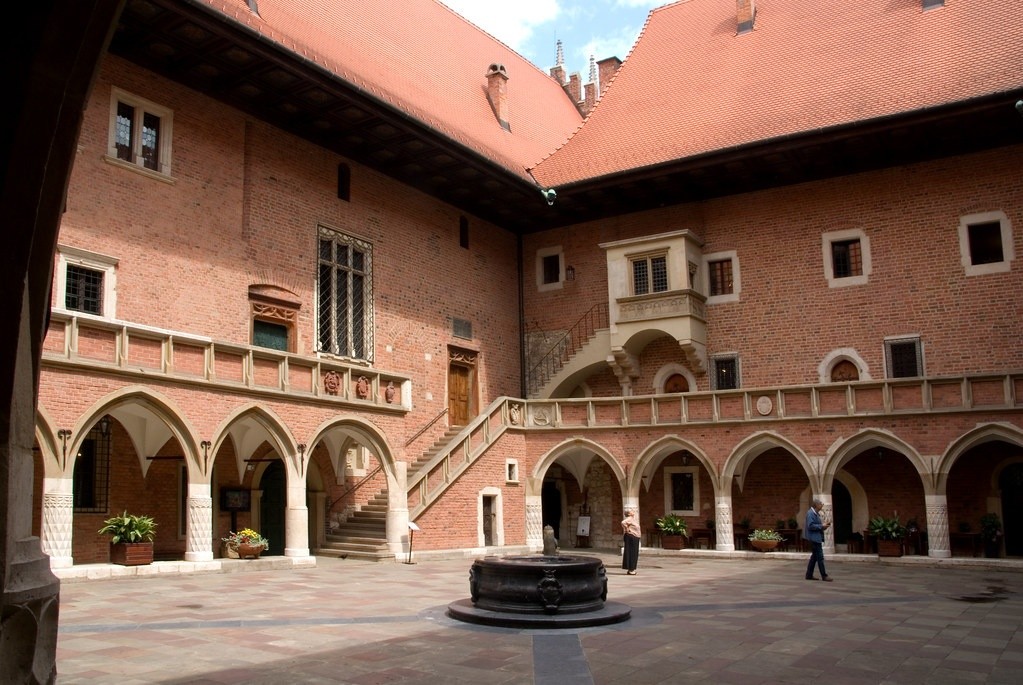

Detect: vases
[230, 542, 265, 559]
[751, 539, 778, 551]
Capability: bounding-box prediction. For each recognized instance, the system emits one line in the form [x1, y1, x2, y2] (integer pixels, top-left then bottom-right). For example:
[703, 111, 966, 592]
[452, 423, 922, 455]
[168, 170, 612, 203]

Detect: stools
[696, 538, 709, 549]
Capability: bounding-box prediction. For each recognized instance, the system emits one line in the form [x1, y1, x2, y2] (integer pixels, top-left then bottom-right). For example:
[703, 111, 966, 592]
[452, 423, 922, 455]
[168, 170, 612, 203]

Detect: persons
[802, 499, 833, 581]
[622, 509, 640, 575]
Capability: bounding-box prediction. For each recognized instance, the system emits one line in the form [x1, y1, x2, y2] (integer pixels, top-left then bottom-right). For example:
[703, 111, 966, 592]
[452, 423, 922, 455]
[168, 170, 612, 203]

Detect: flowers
[220, 526, 268, 548]
[748, 528, 788, 542]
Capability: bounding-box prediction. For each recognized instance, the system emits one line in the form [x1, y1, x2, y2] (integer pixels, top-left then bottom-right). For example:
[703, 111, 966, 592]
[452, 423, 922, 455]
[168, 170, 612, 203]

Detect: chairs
[847, 532, 863, 553]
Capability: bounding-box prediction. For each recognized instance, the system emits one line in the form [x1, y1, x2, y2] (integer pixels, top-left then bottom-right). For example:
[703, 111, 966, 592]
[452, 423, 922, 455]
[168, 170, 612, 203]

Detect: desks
[950, 533, 981, 557]
[775, 528, 802, 552]
[905, 533, 924, 555]
[646, 528, 664, 548]
[732, 526, 753, 550]
[863, 530, 877, 554]
[692, 528, 715, 550]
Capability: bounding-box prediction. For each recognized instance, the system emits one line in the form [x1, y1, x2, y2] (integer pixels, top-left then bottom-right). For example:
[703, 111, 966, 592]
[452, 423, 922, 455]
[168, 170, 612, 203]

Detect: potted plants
[905, 519, 918, 532]
[97, 509, 158, 565]
[739, 516, 751, 530]
[978, 512, 1003, 558]
[655, 513, 691, 550]
[867, 515, 912, 557]
[774, 517, 786, 529]
[705, 520, 716, 528]
[788, 518, 799, 529]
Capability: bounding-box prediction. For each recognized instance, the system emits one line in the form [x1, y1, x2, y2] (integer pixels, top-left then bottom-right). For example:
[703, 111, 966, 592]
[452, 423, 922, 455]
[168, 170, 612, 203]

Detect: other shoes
[805, 576, 819, 580]
[627, 570, 638, 575]
[822, 574, 833, 581]
